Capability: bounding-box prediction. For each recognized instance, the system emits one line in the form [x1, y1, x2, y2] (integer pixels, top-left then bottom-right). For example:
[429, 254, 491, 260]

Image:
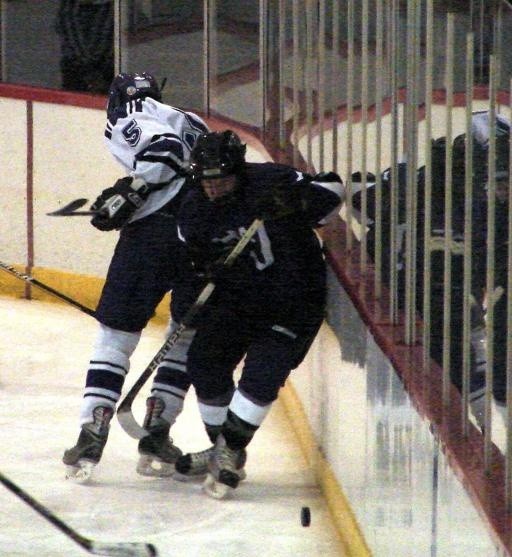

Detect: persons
[54, 0, 151, 93]
[63, 73, 214, 467]
[175, 131, 344, 489]
[345, 110, 510, 438]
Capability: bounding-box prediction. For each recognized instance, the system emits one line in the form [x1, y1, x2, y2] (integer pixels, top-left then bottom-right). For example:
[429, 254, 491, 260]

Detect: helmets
[189, 129, 246, 197]
[106, 70, 162, 119]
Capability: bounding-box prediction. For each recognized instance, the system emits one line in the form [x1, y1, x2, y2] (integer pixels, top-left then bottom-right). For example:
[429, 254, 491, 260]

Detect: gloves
[90, 177, 151, 232]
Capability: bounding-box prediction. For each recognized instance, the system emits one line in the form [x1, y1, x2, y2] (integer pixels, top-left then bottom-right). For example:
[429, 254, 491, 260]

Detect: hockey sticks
[0, 470, 158, 557]
[47, 199, 93, 218]
[116, 189, 272, 441]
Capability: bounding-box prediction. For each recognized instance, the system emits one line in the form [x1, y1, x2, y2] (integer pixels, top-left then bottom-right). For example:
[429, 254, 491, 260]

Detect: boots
[61, 402, 114, 467]
[138, 397, 183, 464]
[208, 432, 243, 489]
[174, 444, 246, 477]
